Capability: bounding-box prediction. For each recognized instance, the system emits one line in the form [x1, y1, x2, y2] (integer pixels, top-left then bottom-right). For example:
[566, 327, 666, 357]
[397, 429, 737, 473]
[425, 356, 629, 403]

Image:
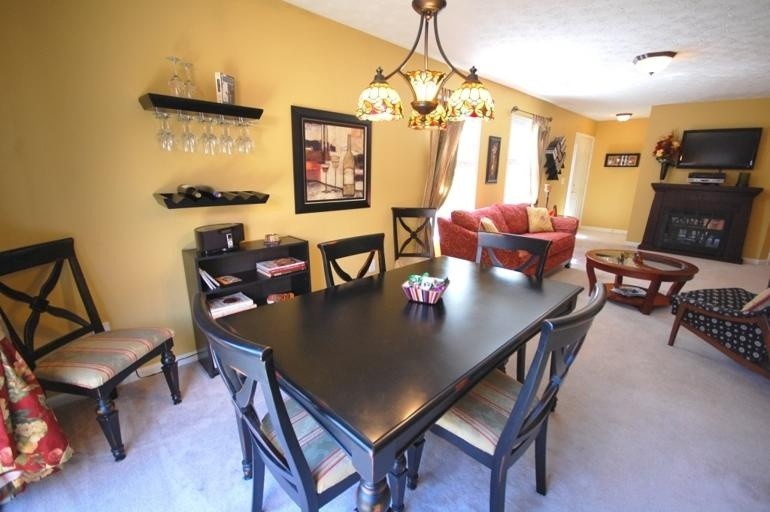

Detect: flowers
[652, 129, 680, 158]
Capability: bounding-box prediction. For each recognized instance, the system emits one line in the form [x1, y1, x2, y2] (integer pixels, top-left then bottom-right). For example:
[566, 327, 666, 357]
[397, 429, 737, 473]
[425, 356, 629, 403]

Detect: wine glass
[331, 157, 341, 192]
[321, 163, 331, 194]
[166, 54, 199, 100]
[151, 110, 257, 157]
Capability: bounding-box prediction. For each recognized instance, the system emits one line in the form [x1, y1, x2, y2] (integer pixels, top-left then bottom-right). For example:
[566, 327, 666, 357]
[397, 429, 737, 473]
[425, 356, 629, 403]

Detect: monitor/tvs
[676, 128, 763, 168]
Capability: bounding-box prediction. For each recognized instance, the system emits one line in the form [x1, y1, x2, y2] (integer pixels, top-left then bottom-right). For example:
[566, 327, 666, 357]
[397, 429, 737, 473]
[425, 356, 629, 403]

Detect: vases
[659, 159, 671, 181]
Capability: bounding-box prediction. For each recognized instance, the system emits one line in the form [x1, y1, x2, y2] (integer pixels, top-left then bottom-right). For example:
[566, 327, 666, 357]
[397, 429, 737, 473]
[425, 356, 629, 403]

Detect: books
[199, 257, 306, 320]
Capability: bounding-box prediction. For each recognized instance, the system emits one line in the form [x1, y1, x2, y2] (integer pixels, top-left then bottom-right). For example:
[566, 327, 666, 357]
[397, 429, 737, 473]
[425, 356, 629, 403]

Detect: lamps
[633, 51, 678, 81]
[616, 113, 632, 123]
[354, 0, 493, 131]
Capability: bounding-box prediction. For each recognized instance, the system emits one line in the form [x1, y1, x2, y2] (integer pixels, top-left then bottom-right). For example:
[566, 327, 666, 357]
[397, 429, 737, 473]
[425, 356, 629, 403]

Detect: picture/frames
[288, 103, 373, 214]
[485, 135, 502, 184]
[604, 152, 640, 168]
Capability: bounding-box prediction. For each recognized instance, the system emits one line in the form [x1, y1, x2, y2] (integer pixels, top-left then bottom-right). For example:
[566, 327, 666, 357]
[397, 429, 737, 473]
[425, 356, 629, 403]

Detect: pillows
[451, 203, 510, 233]
[742, 288, 770, 313]
[481, 216, 498, 232]
[495, 204, 527, 231]
[526, 206, 554, 232]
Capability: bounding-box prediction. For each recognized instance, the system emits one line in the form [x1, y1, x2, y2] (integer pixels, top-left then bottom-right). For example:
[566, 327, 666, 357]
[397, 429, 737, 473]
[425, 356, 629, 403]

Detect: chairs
[195, 293, 408, 511]
[668, 285, 770, 380]
[408, 282, 606, 512]
[318, 232, 386, 285]
[390, 203, 436, 268]
[0, 237, 180, 460]
[477, 226, 552, 384]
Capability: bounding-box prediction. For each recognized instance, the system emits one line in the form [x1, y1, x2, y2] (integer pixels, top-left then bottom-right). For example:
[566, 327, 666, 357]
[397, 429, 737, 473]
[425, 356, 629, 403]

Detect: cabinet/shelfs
[138, 93, 270, 209]
[181, 235, 310, 379]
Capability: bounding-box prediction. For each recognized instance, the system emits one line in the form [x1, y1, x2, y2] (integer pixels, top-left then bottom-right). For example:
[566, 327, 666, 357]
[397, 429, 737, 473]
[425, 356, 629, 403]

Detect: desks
[214, 257, 583, 510]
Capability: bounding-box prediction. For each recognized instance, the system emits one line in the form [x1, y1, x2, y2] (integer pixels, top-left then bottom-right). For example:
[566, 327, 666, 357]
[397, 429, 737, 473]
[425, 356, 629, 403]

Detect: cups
[266, 234, 279, 242]
[736, 171, 751, 188]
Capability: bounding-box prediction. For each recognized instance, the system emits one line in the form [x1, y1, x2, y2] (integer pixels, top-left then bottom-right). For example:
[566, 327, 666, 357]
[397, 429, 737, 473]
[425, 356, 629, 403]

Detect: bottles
[196, 184, 223, 200]
[341, 133, 356, 196]
[177, 183, 201, 199]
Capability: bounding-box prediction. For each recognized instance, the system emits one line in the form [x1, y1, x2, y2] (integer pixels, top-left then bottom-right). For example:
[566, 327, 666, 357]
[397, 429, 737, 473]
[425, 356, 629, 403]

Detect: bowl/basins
[401, 298, 446, 322]
[402, 274, 449, 304]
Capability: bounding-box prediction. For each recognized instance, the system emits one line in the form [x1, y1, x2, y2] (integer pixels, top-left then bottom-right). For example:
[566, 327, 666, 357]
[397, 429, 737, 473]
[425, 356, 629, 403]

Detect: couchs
[436, 203, 579, 276]
